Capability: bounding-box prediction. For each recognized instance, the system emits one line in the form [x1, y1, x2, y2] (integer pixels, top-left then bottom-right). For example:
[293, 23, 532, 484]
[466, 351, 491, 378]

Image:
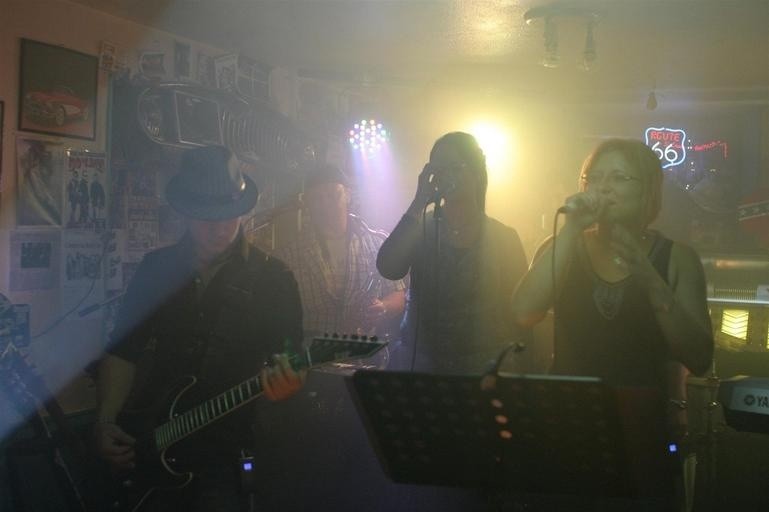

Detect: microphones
[258, 197, 304, 222]
[425, 178, 455, 206]
[559, 200, 587, 218]
[488, 341, 528, 382]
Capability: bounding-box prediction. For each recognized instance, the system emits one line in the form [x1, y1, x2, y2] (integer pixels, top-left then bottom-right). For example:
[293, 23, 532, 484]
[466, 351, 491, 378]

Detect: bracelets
[653, 295, 677, 314]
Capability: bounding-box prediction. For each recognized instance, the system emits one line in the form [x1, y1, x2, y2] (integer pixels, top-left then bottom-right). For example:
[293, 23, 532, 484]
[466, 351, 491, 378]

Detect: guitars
[46, 330, 388, 510]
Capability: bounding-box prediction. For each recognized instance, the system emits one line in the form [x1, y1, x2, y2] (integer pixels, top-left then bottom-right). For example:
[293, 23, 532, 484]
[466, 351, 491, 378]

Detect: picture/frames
[18, 37, 98, 139]
[171, 89, 222, 147]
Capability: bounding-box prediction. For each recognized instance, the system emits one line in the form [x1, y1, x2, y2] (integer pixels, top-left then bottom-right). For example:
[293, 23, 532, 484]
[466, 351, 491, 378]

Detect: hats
[166, 147, 260, 222]
[306, 164, 347, 186]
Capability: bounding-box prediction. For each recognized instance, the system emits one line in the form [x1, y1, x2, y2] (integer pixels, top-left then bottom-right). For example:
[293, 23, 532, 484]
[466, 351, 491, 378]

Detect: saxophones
[339, 214, 391, 369]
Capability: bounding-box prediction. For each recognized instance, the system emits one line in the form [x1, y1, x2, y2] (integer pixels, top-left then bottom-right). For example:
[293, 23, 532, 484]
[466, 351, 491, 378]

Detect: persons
[375, 131, 529, 372]
[505, 134, 715, 403]
[83, 143, 313, 512]
[276, 161, 409, 373]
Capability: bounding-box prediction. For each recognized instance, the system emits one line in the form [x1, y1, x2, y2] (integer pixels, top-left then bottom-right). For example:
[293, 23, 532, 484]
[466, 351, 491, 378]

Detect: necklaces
[613, 254, 623, 266]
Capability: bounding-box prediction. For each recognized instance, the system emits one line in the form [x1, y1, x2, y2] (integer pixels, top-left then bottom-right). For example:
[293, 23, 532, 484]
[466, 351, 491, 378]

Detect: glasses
[581, 172, 642, 184]
